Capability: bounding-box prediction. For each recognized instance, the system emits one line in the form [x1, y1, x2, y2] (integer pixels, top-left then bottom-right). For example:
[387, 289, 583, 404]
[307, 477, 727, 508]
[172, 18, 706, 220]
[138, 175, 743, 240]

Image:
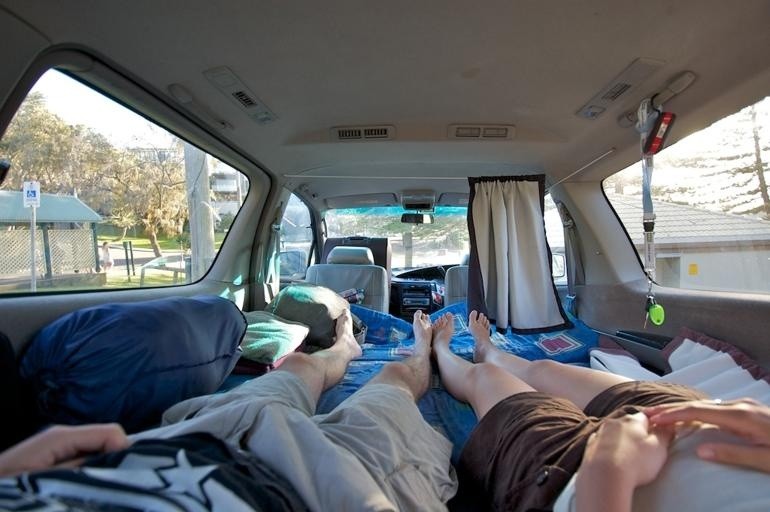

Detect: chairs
[444, 253, 468, 308]
[305, 247, 388, 316]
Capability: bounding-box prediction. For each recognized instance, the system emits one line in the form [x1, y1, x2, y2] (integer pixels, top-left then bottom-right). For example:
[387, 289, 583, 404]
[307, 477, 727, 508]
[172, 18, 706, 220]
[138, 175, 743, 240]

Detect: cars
[279, 248, 309, 291]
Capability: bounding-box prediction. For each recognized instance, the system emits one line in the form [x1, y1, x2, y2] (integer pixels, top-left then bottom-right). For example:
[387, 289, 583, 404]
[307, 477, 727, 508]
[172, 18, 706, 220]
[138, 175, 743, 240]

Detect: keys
[643, 302, 651, 328]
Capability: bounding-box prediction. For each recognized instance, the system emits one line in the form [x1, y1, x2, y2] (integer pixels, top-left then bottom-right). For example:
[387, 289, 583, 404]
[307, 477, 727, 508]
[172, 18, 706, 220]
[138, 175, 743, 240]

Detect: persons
[432, 310, 770, 512]
[0, 307, 458, 512]
[103, 241, 114, 271]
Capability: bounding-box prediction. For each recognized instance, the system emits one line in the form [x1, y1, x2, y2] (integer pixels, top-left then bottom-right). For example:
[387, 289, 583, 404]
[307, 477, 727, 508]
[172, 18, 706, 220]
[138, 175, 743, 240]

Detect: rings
[714, 399, 721, 405]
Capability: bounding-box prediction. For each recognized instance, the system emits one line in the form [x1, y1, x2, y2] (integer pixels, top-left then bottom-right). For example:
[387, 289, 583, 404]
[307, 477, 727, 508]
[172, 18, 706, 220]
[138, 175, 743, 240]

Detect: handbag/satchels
[276, 282, 350, 346]
